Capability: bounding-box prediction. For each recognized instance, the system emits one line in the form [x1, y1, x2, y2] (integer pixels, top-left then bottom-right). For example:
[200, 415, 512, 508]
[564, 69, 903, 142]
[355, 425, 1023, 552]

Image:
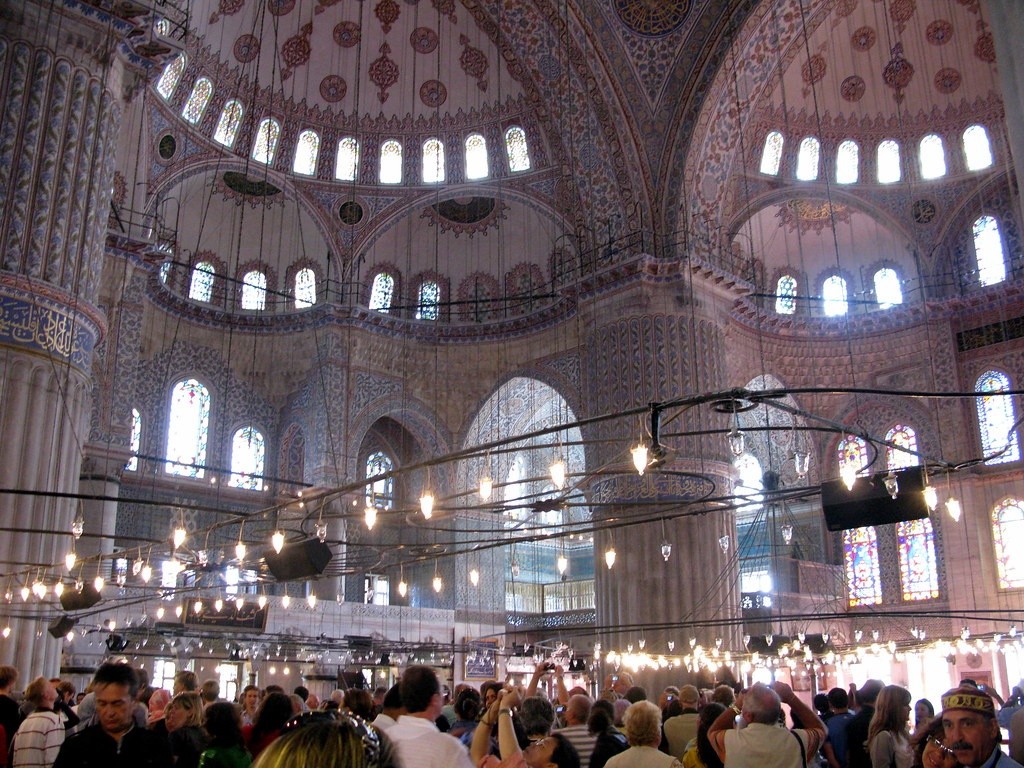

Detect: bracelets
[788, 695, 796, 704]
[498, 709, 513, 717]
[481, 719, 496, 725]
[557, 676, 563, 683]
[729, 704, 741, 715]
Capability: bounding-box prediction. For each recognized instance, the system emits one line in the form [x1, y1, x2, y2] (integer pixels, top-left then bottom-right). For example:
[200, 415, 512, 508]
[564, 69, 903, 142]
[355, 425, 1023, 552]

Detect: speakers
[60, 584, 102, 611]
[48, 617, 75, 638]
[568, 659, 586, 671]
[345, 635, 372, 649]
[821, 466, 930, 532]
[155, 622, 184, 637]
[105, 635, 130, 651]
[744, 634, 832, 655]
[264, 538, 333, 582]
[515, 645, 535, 656]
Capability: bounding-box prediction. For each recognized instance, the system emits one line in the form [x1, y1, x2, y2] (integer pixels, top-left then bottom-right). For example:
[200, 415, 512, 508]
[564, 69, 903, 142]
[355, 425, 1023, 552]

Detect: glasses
[926, 734, 956, 759]
[283, 711, 379, 768]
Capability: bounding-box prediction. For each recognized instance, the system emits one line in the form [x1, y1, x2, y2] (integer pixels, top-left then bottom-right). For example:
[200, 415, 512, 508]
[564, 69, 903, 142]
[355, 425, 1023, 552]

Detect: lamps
[0, 0, 1024, 686]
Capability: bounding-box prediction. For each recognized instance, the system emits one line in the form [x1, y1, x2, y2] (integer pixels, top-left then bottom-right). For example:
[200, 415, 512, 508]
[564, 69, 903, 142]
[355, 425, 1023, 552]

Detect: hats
[941, 687, 995, 716]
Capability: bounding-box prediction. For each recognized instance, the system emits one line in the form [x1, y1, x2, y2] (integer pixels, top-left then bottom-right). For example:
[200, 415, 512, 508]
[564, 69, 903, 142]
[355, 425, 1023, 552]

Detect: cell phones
[544, 663, 555, 670]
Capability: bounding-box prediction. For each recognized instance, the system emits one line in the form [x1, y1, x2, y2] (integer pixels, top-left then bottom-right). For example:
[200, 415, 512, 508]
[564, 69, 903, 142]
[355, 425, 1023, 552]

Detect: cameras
[555, 706, 566, 713]
[612, 676, 619, 681]
[977, 683, 986, 692]
[667, 694, 673, 701]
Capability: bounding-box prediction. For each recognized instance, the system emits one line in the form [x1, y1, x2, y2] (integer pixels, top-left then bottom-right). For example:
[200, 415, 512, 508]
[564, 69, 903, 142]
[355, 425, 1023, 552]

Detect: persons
[1, 659, 1024, 768]
